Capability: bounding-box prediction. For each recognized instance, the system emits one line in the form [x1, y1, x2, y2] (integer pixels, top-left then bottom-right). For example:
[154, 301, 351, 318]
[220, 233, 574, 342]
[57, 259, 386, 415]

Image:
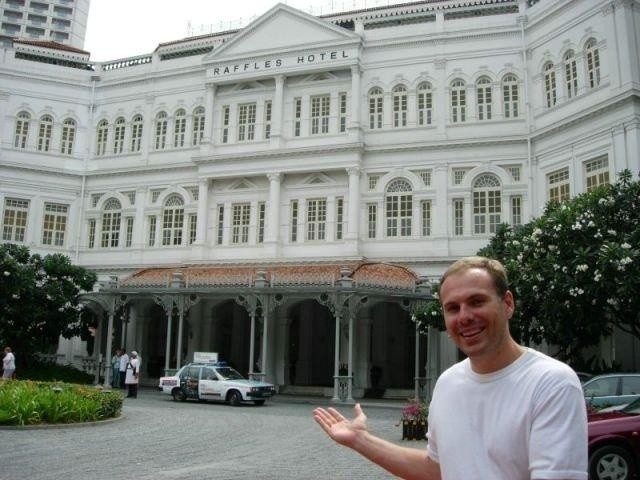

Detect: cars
[158, 361, 274, 407]
[577, 371, 640, 480]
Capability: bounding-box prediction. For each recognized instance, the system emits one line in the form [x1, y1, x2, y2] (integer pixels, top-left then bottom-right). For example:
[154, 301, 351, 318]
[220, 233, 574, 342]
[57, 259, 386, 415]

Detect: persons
[2, 347, 16, 378]
[314, 256, 589, 480]
[112, 348, 143, 400]
